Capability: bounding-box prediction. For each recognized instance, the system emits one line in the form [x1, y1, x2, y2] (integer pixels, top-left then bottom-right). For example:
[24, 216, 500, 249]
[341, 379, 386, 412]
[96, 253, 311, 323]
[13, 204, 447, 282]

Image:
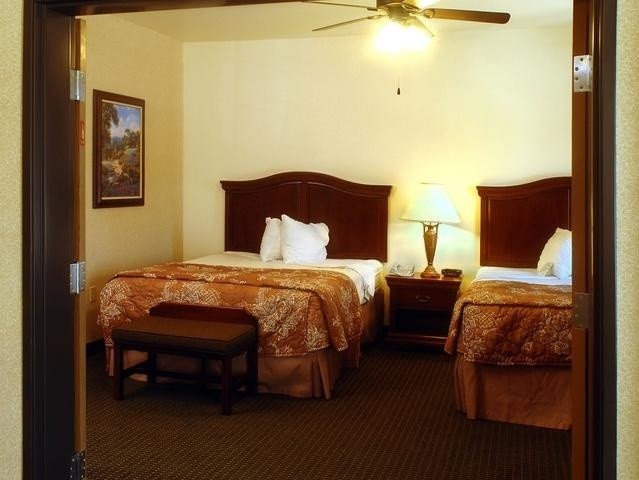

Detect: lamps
[399, 182, 462, 278]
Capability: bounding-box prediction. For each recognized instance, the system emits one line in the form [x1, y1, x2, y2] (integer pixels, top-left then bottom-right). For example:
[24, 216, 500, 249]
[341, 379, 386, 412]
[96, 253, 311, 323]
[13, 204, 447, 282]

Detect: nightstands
[385, 271, 463, 349]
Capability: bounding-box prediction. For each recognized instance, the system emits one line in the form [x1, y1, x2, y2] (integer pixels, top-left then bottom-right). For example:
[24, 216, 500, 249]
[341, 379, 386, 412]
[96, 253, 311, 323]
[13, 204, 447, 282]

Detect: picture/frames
[92, 90, 145, 209]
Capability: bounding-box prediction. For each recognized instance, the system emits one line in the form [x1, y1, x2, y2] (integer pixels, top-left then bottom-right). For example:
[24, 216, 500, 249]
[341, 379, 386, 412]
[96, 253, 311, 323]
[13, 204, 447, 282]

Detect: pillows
[536, 227, 572, 283]
[260, 215, 330, 265]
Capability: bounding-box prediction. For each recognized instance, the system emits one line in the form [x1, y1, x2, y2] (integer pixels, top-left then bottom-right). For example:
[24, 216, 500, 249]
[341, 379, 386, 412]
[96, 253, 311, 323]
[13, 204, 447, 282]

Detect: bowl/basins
[395, 264, 416, 277]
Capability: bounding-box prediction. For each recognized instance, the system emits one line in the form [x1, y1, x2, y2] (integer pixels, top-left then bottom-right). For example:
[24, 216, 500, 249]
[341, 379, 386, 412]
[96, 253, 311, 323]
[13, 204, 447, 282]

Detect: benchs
[111, 316, 259, 416]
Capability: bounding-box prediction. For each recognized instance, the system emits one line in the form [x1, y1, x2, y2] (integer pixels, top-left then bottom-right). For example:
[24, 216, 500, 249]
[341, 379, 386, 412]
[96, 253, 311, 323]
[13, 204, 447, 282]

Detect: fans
[303, 0, 511, 33]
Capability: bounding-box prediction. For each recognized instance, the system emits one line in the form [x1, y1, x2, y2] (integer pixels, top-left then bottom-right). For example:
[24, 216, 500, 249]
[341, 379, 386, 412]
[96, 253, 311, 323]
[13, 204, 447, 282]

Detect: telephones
[389, 263, 415, 277]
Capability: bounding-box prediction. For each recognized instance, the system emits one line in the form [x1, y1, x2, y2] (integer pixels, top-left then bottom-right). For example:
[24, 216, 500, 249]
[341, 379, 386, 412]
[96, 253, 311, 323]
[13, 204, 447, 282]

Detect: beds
[456, 176, 572, 430]
[103, 172, 392, 400]
